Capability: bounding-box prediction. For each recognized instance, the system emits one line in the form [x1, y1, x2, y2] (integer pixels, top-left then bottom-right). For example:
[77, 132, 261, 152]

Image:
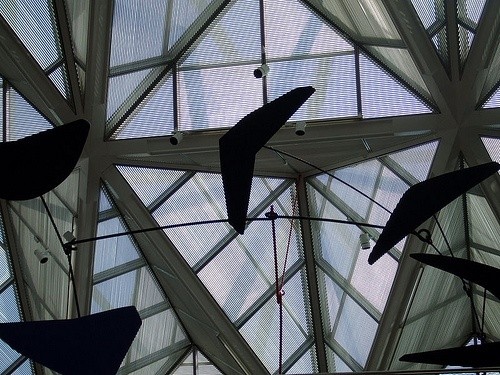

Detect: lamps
[359, 233, 372, 250]
[171, 132, 184, 147]
[33, 248, 50, 264]
[294, 121, 306, 138]
[254, 64, 270, 79]
[63, 230, 76, 245]
